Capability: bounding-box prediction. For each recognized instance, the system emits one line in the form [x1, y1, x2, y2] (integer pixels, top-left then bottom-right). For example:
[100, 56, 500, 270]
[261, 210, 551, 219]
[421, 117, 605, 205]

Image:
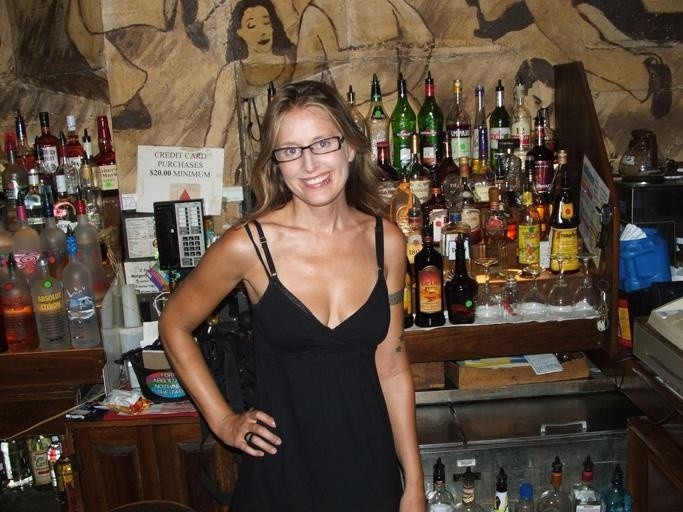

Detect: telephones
[154, 199, 207, 275]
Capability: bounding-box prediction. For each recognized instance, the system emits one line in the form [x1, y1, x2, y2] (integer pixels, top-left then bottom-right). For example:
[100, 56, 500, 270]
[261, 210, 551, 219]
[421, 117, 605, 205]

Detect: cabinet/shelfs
[623, 421, 683, 511]
[70, 173, 616, 507]
[1, 345, 106, 444]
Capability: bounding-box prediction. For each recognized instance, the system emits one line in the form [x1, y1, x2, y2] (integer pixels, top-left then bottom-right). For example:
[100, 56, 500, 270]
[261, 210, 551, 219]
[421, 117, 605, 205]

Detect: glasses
[271, 137, 345, 163]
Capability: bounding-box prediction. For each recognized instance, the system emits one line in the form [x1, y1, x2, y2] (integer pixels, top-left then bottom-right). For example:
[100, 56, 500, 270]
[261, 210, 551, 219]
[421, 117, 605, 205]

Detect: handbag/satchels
[200, 317, 256, 414]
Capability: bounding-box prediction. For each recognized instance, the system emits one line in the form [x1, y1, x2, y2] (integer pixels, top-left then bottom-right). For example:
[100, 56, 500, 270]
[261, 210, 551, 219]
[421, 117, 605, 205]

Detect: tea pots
[617, 128, 667, 183]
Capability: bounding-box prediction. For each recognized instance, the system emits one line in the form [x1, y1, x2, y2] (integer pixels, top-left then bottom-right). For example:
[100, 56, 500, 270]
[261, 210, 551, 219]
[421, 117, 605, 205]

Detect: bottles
[0, 111, 118, 350]
[346, 70, 580, 328]
[456, 465, 486, 512]
[516, 483, 534, 512]
[427, 457, 455, 512]
[537, 455, 574, 512]
[602, 464, 634, 512]
[26, 430, 80, 511]
[489, 467, 512, 512]
[569, 454, 606, 512]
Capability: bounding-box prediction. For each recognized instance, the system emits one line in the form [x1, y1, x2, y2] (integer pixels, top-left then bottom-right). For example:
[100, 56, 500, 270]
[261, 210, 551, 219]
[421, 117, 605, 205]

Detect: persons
[159, 80, 426, 512]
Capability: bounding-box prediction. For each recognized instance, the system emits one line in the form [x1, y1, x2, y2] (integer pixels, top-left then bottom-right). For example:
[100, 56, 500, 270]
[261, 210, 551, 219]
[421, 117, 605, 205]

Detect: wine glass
[471, 253, 602, 322]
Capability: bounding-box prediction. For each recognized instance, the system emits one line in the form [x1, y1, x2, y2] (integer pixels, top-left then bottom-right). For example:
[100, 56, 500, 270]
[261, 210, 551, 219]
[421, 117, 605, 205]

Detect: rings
[244, 432, 254, 443]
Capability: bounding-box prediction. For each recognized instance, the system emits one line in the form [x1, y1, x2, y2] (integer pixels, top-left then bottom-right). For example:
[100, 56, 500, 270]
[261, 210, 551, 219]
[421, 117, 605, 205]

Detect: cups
[0, 438, 33, 490]
